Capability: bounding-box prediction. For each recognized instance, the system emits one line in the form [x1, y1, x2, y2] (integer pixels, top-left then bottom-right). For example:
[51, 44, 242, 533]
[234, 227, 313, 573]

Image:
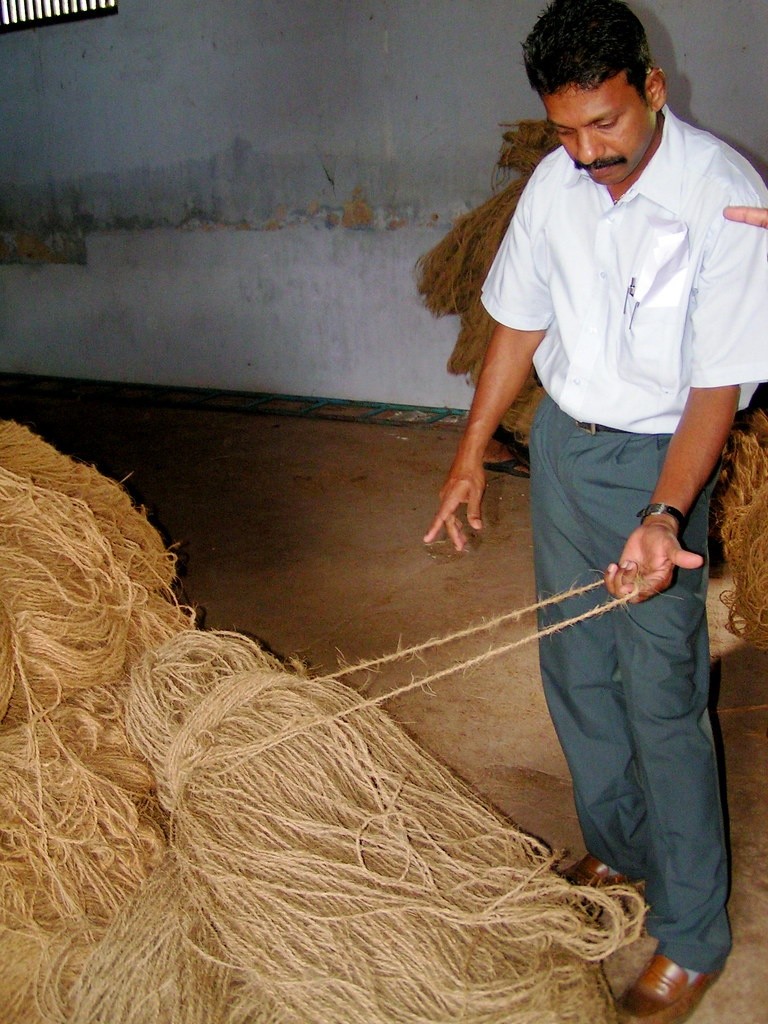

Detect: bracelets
[635, 502, 685, 533]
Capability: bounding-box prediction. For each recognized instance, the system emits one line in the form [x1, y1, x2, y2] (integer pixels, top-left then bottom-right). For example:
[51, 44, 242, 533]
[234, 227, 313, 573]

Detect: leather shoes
[614, 942, 718, 1024]
[561, 854, 632, 890]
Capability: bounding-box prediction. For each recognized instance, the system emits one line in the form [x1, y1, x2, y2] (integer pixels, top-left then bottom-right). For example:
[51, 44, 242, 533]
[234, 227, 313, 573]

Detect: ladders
[0, 370, 470, 429]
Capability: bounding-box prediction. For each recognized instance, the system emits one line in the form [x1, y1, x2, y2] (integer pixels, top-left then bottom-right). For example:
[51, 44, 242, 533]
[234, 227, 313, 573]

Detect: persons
[424, 0, 768, 1024]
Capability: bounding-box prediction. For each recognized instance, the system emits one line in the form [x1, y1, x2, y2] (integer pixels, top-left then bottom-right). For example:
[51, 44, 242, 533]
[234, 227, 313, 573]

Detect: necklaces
[613, 192, 624, 206]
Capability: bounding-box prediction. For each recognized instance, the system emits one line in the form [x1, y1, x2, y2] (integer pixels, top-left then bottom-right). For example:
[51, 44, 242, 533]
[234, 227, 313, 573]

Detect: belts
[575, 421, 629, 434]
